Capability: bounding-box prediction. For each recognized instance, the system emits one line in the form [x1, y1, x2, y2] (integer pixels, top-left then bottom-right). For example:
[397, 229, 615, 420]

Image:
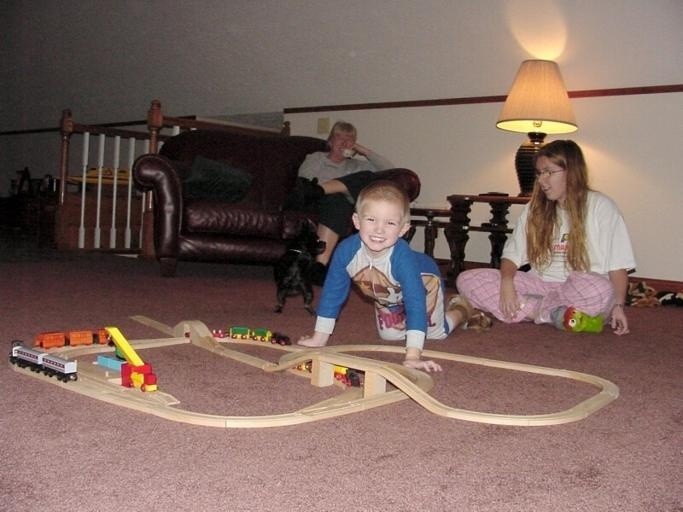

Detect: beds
[50, 100, 289, 264]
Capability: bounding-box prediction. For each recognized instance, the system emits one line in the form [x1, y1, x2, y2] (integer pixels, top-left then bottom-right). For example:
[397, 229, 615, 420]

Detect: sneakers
[290, 173, 325, 212]
[445, 294, 495, 331]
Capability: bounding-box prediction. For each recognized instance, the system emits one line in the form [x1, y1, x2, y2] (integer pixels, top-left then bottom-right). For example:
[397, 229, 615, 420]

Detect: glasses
[533, 170, 566, 178]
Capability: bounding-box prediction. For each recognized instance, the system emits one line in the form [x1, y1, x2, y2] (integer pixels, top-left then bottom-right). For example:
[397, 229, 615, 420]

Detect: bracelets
[615, 303, 624, 308]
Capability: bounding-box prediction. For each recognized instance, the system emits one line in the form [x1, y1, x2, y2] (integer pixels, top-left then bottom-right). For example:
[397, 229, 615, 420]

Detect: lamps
[494, 60, 579, 196]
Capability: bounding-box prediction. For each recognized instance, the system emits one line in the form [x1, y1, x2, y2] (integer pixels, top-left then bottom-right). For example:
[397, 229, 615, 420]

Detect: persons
[297, 121, 397, 287]
[456, 140, 637, 336]
[299, 178, 477, 373]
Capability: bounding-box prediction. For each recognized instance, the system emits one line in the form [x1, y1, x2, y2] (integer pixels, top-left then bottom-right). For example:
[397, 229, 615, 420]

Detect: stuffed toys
[552, 306, 606, 334]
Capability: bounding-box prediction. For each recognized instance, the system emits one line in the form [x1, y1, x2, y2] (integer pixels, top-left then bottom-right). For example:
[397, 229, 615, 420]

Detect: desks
[444, 195, 534, 293]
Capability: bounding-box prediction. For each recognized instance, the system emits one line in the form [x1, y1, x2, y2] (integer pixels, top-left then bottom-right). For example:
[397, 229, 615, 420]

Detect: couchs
[130, 131, 422, 278]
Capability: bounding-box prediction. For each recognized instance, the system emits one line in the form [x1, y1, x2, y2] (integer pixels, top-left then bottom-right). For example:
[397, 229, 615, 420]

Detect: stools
[406, 209, 455, 287]
